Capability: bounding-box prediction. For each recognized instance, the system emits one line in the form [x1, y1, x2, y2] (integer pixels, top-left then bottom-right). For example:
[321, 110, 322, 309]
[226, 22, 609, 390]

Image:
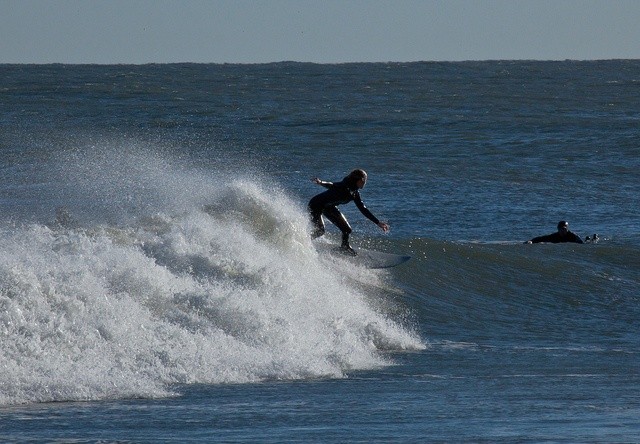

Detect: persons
[304, 166, 390, 258]
[520, 218, 585, 246]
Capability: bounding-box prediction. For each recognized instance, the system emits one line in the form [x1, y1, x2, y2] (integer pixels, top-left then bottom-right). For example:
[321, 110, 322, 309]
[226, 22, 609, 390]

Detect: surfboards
[310, 240, 410, 269]
